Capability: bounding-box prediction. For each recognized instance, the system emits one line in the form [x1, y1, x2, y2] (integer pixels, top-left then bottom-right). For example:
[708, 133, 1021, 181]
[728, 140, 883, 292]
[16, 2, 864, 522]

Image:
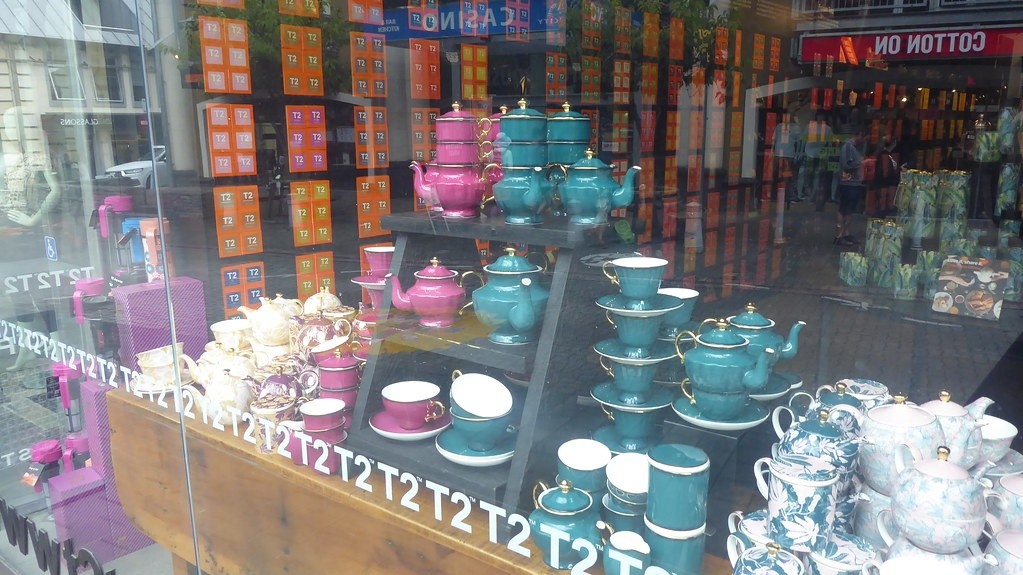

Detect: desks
[731, 264, 1023, 451]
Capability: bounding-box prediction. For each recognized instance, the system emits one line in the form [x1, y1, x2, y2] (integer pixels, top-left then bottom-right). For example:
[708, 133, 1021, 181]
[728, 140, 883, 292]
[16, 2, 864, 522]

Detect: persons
[771, 108, 832, 205]
[832, 125, 878, 246]
[871, 133, 908, 218]
[1, 105, 62, 373]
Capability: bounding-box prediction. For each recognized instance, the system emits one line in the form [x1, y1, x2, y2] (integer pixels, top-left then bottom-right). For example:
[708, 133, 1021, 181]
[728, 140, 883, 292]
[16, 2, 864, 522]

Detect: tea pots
[675, 302, 807, 393]
[826, 376, 1023, 575]
[197, 285, 354, 426]
[386, 96, 642, 346]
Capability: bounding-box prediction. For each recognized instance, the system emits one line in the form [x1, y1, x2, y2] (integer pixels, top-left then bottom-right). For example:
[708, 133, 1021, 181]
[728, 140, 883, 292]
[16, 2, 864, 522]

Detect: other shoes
[759, 196, 778, 202]
[790, 198, 803, 204]
[845, 235, 861, 244]
[834, 237, 853, 245]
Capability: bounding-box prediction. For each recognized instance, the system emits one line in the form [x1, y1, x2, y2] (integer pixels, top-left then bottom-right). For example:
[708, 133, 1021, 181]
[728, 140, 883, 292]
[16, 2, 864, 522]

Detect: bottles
[862, 109, 1023, 289]
[531, 480, 614, 568]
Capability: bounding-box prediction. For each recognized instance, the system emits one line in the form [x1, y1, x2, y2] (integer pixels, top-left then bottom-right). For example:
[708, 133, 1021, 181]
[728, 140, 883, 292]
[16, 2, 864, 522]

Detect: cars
[103, 146, 169, 192]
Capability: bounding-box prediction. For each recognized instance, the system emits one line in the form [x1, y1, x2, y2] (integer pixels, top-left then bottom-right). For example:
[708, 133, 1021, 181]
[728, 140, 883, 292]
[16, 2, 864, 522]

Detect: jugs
[35, 196, 175, 492]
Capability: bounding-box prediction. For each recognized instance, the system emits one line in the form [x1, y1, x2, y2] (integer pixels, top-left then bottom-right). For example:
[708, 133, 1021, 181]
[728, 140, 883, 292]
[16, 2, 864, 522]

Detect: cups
[955, 295, 965, 304]
[593, 255, 700, 403]
[380, 368, 513, 453]
[362, 244, 394, 354]
[978, 414, 1017, 466]
[135, 341, 186, 385]
[554, 439, 709, 574]
[838, 218, 1023, 300]
[280, 398, 348, 475]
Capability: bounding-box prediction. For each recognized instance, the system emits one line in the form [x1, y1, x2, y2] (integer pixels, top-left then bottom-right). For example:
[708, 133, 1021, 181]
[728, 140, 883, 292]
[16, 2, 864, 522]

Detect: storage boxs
[660, 391, 788, 557]
[46, 276, 208, 571]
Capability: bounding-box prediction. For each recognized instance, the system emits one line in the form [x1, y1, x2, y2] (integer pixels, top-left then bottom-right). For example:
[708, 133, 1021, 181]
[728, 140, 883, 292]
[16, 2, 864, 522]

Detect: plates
[932, 259, 1004, 319]
[370, 410, 520, 469]
[588, 321, 803, 457]
[130, 369, 196, 394]
[970, 448, 1022, 477]
[351, 276, 386, 361]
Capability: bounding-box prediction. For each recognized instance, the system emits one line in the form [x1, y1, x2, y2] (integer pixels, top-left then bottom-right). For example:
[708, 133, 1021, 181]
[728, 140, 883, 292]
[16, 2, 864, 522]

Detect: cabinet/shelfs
[337, 211, 636, 545]
